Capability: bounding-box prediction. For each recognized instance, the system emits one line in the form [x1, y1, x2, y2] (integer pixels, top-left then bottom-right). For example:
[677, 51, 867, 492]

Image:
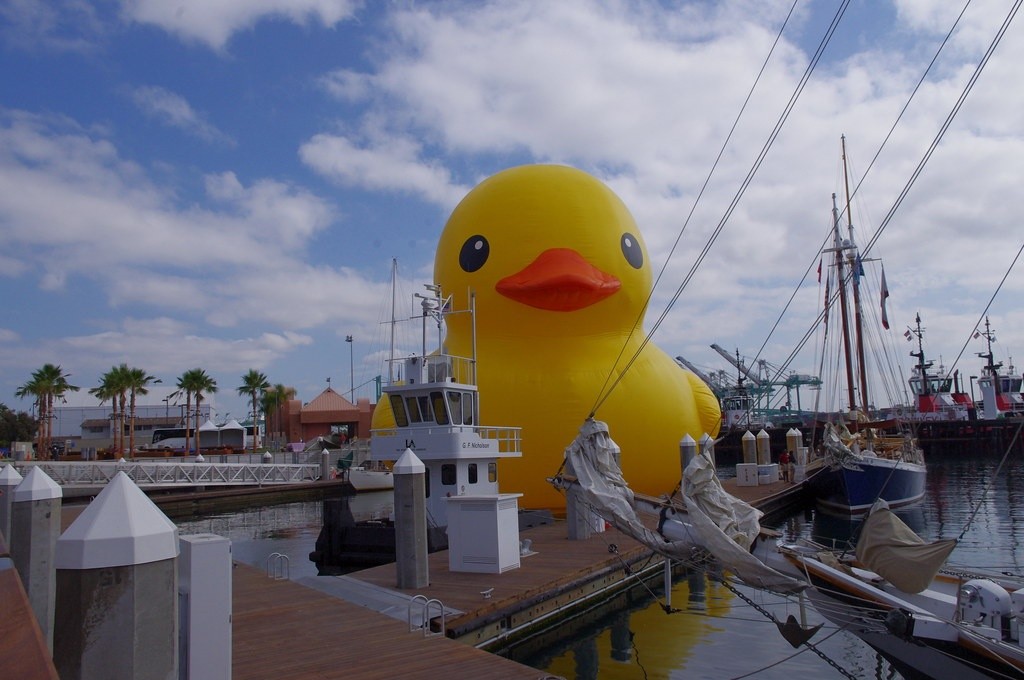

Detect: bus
[150, 428, 196, 453]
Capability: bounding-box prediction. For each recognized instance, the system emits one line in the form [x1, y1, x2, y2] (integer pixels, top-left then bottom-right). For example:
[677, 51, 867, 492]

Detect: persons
[298, 437, 302, 443]
[340, 433, 346, 444]
[317, 433, 325, 452]
[353, 435, 357, 443]
[779, 448, 797, 484]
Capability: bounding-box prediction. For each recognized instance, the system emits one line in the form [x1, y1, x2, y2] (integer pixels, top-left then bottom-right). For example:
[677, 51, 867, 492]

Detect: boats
[309, 278, 552, 575]
[887, 314, 1024, 441]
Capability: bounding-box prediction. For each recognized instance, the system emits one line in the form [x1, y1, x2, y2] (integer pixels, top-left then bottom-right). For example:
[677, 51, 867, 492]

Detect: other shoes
[791, 482, 798, 484]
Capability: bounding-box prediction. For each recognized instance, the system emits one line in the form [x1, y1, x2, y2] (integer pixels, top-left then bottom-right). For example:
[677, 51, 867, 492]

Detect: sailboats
[560, 0, 1024, 680]
[802, 137, 931, 522]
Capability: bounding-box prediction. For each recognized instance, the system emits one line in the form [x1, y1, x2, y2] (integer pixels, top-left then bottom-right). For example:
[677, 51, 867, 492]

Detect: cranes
[676, 343, 820, 423]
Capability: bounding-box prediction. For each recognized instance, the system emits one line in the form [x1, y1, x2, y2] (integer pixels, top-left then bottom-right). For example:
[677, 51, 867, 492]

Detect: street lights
[161, 399, 168, 425]
[345, 333, 354, 404]
[179, 404, 187, 428]
[33, 403, 39, 441]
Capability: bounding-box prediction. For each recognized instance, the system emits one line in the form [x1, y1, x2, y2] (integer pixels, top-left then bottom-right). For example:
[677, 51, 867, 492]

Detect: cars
[33, 442, 70, 458]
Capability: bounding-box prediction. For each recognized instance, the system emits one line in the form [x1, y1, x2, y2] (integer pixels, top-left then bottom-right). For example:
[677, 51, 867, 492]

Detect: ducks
[369, 164, 722, 515]
